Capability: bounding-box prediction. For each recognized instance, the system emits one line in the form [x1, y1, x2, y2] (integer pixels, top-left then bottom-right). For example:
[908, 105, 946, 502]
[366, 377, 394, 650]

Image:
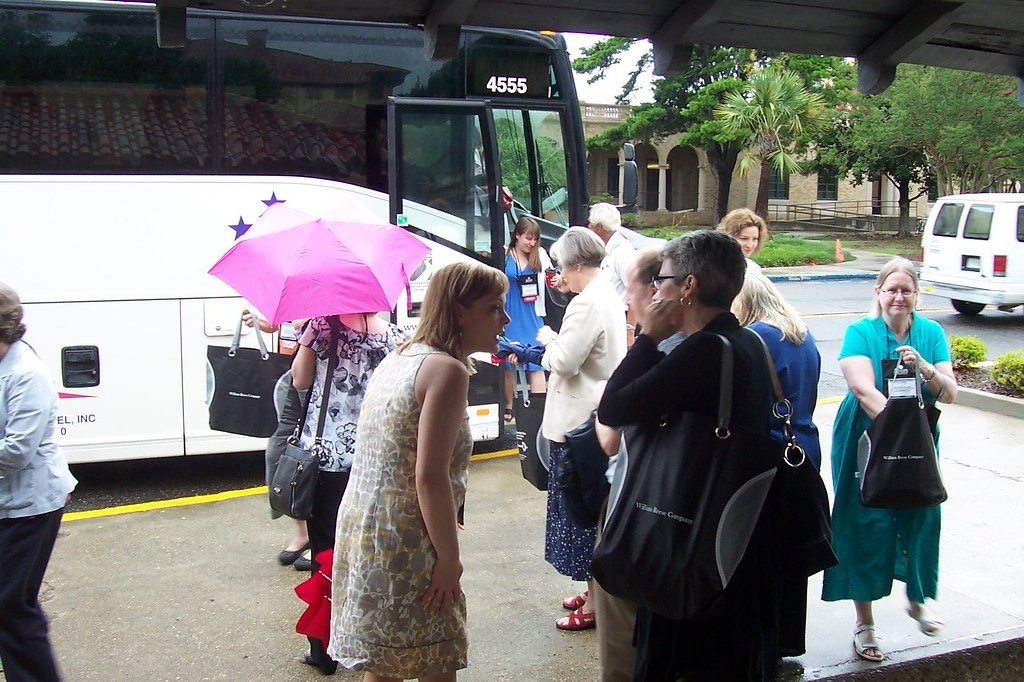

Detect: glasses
[650, 275, 676, 291]
[879, 287, 918, 298]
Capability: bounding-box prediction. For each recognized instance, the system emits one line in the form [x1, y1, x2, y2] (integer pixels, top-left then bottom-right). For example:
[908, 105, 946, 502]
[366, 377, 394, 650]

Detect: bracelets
[920, 369, 938, 383]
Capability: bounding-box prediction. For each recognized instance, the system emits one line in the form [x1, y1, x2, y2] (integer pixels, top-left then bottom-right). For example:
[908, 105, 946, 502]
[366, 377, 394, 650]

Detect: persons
[241, 309, 313, 572]
[472, 126, 490, 217]
[497, 217, 554, 421]
[818, 257, 957, 662]
[288, 310, 411, 676]
[536, 204, 840, 682]
[0, 282, 80, 682]
[321, 260, 512, 682]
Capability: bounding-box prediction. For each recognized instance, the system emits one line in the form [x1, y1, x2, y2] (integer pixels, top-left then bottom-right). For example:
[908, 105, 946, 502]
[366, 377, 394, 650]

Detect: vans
[917, 193, 1024, 315]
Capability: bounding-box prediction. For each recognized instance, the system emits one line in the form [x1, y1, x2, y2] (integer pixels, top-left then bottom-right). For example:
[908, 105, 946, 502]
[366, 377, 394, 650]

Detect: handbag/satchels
[204, 312, 295, 439]
[509, 361, 550, 491]
[857, 351, 949, 505]
[585, 328, 837, 601]
[269, 444, 320, 520]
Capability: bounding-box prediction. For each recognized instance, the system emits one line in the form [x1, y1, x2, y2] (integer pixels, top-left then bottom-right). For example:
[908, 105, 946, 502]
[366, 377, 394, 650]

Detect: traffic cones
[835, 238, 846, 261]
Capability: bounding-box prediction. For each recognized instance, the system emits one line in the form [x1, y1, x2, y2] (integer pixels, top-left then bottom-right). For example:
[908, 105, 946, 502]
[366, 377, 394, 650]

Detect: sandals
[554, 606, 597, 632]
[852, 621, 884, 660]
[563, 589, 590, 611]
[909, 608, 945, 636]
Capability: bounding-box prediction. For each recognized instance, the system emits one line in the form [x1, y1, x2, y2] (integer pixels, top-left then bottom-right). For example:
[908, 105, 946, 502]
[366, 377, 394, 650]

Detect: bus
[1, 0, 638, 466]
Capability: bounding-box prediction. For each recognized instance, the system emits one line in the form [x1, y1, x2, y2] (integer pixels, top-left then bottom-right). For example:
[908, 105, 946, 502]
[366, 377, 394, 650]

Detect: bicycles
[914, 217, 927, 236]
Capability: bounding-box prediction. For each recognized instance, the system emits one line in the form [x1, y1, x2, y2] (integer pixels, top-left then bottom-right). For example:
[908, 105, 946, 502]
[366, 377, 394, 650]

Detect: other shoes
[294, 550, 310, 571]
[504, 408, 513, 420]
[279, 541, 311, 563]
[304, 648, 316, 666]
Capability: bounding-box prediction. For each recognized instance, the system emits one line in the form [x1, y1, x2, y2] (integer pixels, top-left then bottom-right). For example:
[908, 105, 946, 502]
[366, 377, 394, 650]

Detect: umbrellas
[495, 341, 546, 365]
[205, 207, 436, 334]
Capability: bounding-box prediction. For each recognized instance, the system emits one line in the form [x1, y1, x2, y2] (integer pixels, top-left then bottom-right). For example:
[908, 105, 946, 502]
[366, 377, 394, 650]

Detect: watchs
[626, 323, 636, 330]
